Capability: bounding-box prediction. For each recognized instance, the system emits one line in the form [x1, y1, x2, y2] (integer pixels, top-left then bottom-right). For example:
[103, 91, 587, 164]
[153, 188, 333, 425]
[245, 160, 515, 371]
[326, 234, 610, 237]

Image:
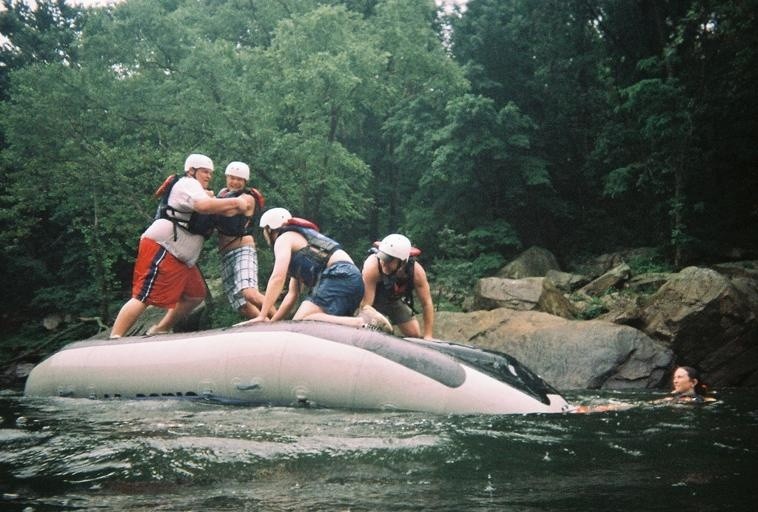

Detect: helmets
[259, 207, 292, 230]
[184, 154, 214, 173]
[378, 234, 411, 263]
[225, 162, 250, 181]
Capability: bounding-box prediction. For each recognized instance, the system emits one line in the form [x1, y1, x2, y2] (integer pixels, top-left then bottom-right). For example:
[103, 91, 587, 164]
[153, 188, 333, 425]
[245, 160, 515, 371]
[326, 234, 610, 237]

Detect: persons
[359, 234, 443, 343]
[206, 160, 276, 322]
[109, 152, 250, 339]
[568, 365, 718, 414]
[232, 207, 394, 335]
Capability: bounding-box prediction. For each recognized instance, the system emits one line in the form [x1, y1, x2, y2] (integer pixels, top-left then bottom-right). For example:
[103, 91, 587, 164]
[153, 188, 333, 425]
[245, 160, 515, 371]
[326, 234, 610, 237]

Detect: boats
[24, 318, 571, 421]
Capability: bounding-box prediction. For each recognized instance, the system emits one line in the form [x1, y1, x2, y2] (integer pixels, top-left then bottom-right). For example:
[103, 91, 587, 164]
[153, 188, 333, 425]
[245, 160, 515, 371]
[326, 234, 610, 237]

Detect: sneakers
[358, 305, 393, 333]
